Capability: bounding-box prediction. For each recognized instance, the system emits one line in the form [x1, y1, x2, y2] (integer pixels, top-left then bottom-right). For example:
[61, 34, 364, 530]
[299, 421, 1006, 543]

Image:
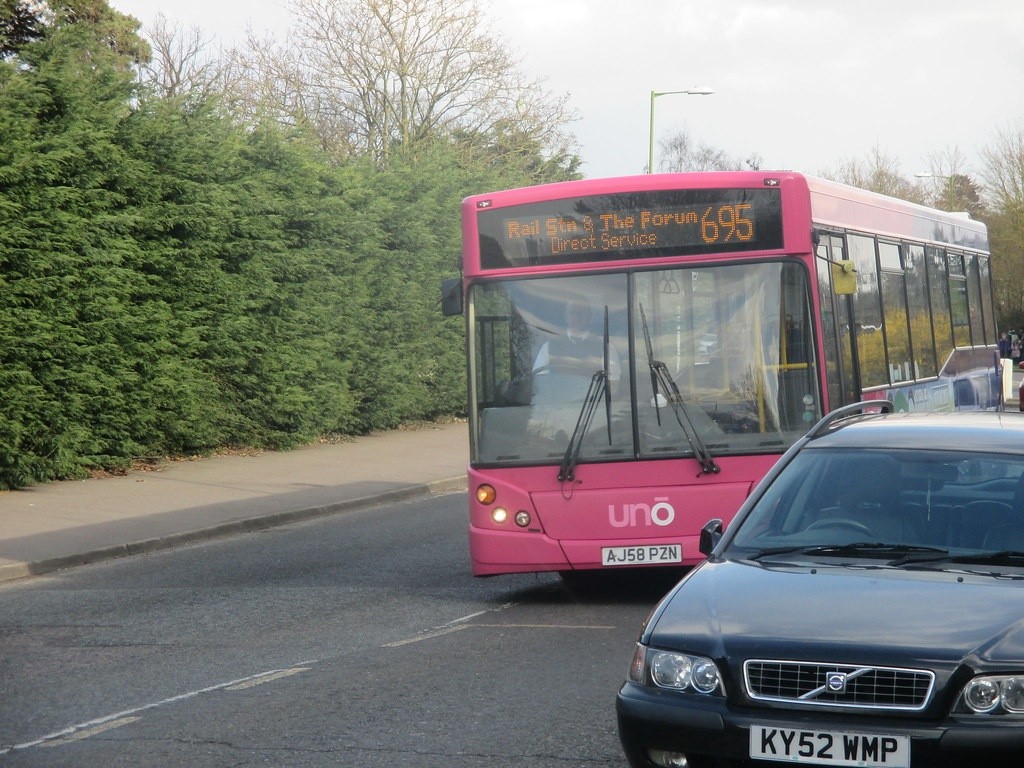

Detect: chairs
[813, 456, 1024, 555]
[711, 310, 877, 415]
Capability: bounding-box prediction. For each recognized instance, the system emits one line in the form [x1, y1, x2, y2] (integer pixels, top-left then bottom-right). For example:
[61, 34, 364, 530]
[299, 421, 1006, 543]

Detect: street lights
[913, 172, 957, 213]
[647, 91, 713, 172]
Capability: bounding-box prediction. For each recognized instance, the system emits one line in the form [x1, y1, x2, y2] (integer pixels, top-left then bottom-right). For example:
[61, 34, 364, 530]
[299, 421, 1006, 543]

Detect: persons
[999, 332, 1024, 366]
[532, 294, 621, 397]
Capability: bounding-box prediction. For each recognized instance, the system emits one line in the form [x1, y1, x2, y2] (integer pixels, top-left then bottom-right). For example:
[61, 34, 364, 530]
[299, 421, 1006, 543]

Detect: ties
[572, 337, 582, 344]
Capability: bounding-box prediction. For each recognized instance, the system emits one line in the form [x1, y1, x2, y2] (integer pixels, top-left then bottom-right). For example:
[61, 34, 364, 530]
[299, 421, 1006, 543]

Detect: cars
[697, 333, 717, 355]
[608, 400, 1024, 768]
[1017, 361, 1024, 411]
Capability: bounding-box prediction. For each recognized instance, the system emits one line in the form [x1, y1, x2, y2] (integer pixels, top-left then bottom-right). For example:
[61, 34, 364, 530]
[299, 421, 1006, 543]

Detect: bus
[440, 171, 1003, 593]
